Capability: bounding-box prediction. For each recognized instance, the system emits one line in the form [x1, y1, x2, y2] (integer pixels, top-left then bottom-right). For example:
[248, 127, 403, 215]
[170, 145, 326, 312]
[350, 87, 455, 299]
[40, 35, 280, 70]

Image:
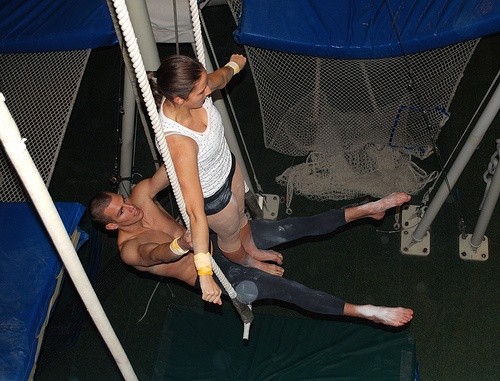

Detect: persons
[90, 164, 413, 327]
[147, 55, 285, 305]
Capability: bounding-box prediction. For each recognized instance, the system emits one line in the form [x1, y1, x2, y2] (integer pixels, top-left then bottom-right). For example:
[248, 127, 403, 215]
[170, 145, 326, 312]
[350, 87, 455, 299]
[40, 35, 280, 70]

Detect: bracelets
[170, 237, 189, 255]
[194, 253, 213, 275]
[224, 61, 240, 75]
